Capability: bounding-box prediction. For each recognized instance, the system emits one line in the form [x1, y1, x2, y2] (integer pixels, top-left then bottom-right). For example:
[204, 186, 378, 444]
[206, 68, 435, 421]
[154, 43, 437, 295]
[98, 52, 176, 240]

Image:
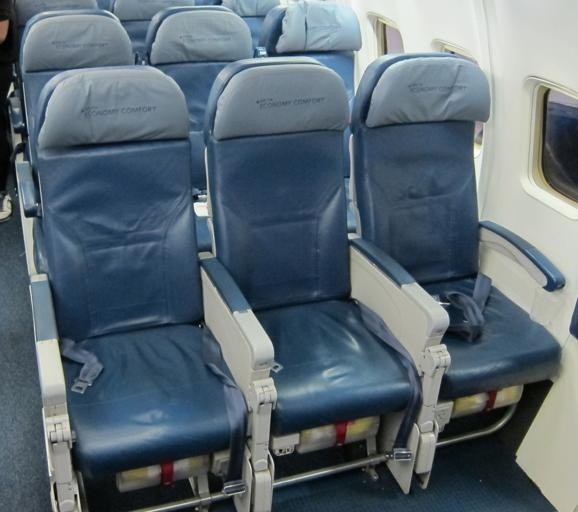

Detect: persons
[0, 0, 19, 224]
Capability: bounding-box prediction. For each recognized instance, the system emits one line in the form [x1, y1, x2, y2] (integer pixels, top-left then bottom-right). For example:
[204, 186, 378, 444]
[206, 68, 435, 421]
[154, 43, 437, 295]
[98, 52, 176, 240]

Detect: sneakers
[1, 192, 13, 223]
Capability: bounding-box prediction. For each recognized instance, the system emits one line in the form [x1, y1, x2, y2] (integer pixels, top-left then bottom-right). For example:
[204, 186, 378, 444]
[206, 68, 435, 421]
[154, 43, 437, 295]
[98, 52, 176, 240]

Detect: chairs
[9, 0, 565, 512]
